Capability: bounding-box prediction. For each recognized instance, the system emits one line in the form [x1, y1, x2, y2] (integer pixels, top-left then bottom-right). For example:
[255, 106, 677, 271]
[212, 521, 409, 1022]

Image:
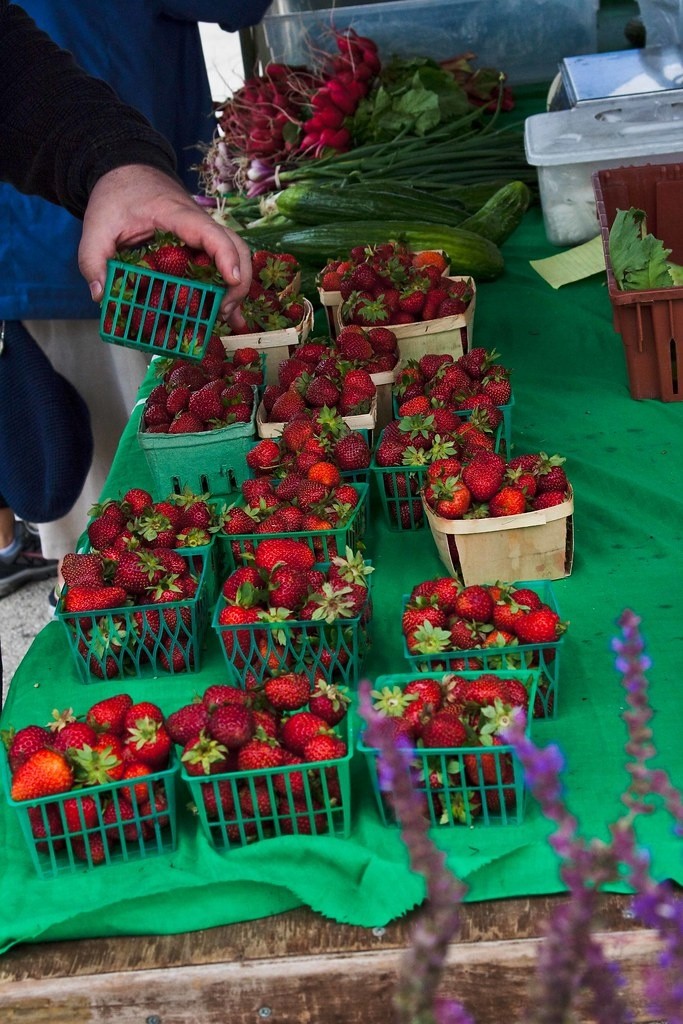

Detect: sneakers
[0, 519, 59, 598]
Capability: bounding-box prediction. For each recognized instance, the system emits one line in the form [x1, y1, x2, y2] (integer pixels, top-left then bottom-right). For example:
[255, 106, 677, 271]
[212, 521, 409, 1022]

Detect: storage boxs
[261, 1, 602, 90]
[517, 89, 683, 244]
[592, 162, 683, 404]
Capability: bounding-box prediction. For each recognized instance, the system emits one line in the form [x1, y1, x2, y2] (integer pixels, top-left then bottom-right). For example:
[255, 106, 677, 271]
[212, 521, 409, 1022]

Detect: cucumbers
[236, 180, 531, 306]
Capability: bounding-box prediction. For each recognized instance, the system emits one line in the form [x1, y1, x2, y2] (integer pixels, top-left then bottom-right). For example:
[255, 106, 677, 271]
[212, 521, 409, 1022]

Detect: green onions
[186, 74, 539, 227]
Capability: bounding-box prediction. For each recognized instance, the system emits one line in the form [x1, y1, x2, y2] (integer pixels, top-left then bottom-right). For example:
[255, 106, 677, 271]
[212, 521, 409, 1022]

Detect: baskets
[0, 249, 577, 876]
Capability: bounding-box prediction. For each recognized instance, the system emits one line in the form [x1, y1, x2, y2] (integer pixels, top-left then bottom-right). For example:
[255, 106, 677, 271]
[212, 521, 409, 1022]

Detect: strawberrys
[8, 240, 569, 829]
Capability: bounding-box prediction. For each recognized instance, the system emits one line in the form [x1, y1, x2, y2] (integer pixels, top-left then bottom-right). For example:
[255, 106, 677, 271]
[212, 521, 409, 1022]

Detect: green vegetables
[608, 205, 683, 289]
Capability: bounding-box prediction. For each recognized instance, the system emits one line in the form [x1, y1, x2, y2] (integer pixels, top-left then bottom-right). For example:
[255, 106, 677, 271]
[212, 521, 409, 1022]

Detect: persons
[0, 0, 273, 601]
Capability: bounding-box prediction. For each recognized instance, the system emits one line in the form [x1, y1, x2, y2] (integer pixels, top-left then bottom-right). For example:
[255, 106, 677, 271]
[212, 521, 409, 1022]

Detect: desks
[0, 206, 683, 1024]
